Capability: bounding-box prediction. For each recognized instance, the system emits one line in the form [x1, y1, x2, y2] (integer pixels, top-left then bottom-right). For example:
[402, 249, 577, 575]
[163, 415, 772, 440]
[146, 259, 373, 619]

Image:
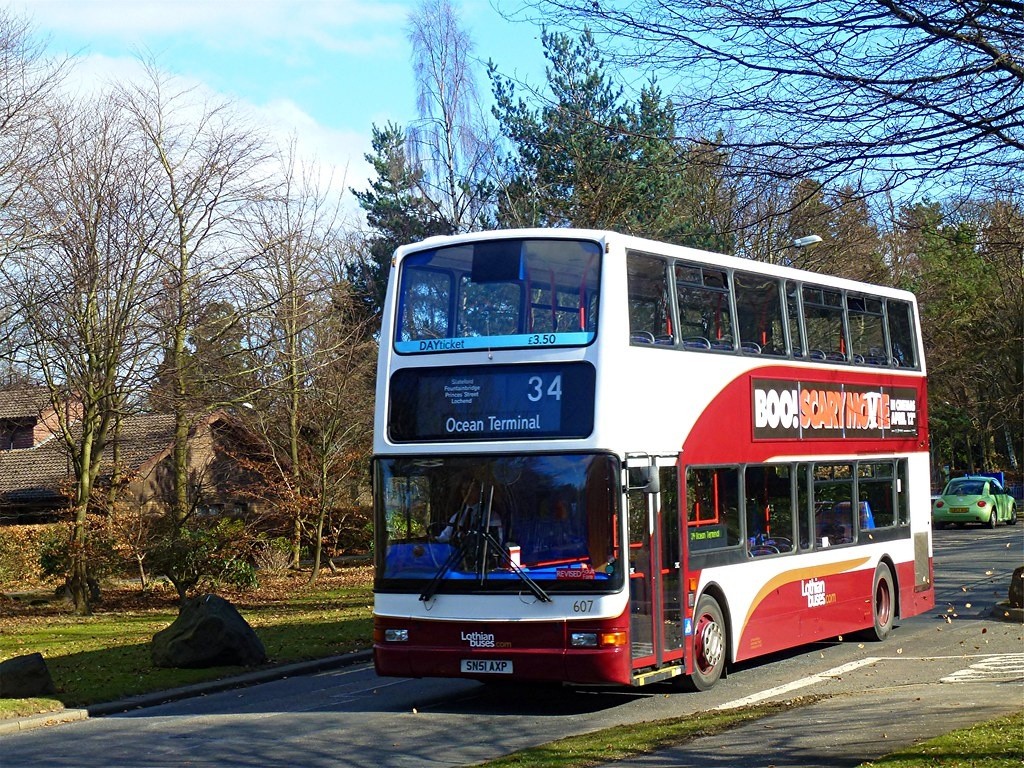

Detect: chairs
[631, 330, 901, 369]
[751, 533, 796, 554]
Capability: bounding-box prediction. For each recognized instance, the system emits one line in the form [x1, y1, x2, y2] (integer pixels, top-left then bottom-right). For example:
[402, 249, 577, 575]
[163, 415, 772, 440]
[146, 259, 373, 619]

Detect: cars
[933, 476, 1018, 530]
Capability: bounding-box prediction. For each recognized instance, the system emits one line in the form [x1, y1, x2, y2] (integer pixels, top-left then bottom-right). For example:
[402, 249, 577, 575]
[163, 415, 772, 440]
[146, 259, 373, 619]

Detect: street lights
[763, 234, 823, 263]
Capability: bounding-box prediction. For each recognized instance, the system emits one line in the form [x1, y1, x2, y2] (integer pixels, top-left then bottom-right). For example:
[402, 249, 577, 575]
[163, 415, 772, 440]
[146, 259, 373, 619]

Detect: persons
[427, 476, 503, 573]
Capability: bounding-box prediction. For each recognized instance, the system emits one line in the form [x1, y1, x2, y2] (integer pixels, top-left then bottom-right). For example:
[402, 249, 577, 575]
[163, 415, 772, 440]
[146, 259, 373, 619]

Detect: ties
[464, 508, 473, 526]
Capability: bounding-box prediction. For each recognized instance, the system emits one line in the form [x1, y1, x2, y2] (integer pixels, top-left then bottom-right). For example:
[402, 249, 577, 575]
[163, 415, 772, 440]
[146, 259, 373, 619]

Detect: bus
[369, 228, 938, 692]
